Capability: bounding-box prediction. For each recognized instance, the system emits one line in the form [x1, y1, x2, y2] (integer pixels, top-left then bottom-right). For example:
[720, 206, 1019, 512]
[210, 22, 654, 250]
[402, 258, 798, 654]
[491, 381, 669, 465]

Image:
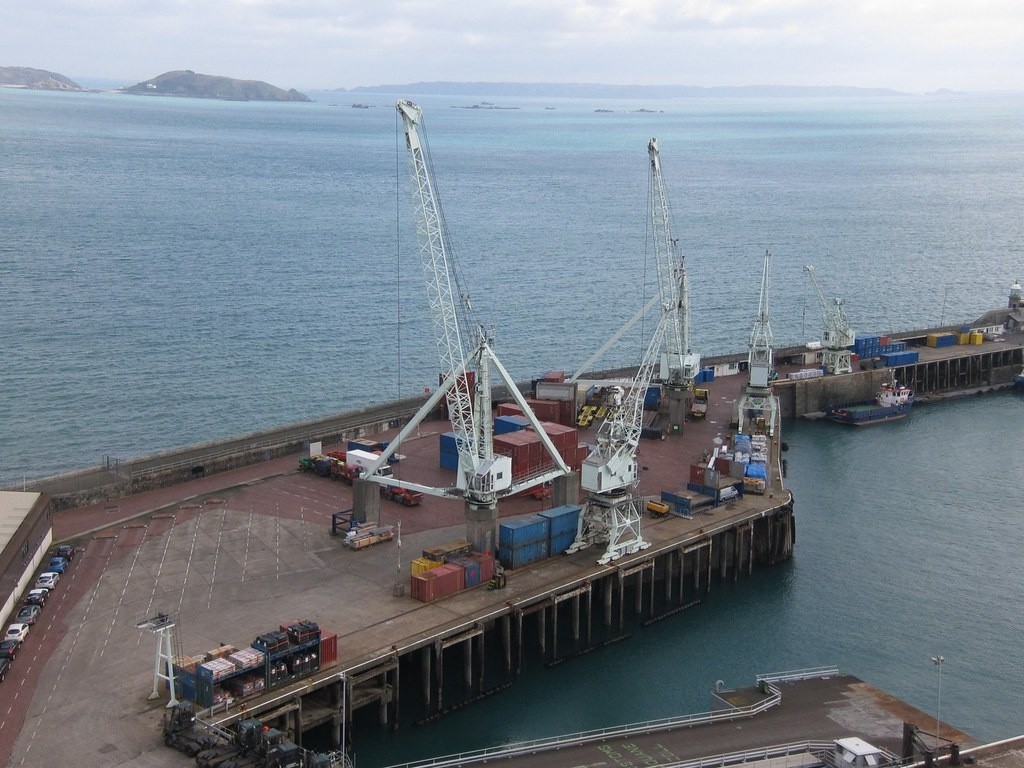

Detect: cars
[0, 545, 80, 682]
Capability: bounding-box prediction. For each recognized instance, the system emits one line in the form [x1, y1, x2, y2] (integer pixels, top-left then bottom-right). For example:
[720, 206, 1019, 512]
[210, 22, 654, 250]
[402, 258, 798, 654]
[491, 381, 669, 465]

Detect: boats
[823, 366, 931, 436]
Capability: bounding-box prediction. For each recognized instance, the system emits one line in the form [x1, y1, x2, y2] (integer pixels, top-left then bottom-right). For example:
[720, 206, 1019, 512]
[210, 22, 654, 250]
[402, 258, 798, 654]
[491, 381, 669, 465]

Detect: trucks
[346, 449, 394, 480]
[691, 387, 721, 420]
[349, 439, 400, 465]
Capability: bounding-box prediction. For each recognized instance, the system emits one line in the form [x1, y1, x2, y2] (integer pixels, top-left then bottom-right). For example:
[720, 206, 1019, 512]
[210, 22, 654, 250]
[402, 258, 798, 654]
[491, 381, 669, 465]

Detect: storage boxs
[855, 335, 920, 368]
[410, 504, 588, 602]
[167, 618, 337, 709]
[437, 371, 590, 497]
[926, 331, 983, 348]
[696, 369, 715, 384]
[346, 438, 382, 468]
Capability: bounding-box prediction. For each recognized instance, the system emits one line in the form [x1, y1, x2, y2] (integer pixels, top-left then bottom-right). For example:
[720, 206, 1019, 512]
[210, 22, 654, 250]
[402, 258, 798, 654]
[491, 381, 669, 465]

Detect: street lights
[930, 654, 945, 767]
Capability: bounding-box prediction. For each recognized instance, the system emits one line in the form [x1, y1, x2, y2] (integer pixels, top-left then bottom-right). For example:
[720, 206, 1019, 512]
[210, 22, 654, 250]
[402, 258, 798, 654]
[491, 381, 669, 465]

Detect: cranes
[726, 247, 783, 438]
[802, 263, 863, 376]
[564, 130, 705, 564]
[355, 83, 573, 583]
